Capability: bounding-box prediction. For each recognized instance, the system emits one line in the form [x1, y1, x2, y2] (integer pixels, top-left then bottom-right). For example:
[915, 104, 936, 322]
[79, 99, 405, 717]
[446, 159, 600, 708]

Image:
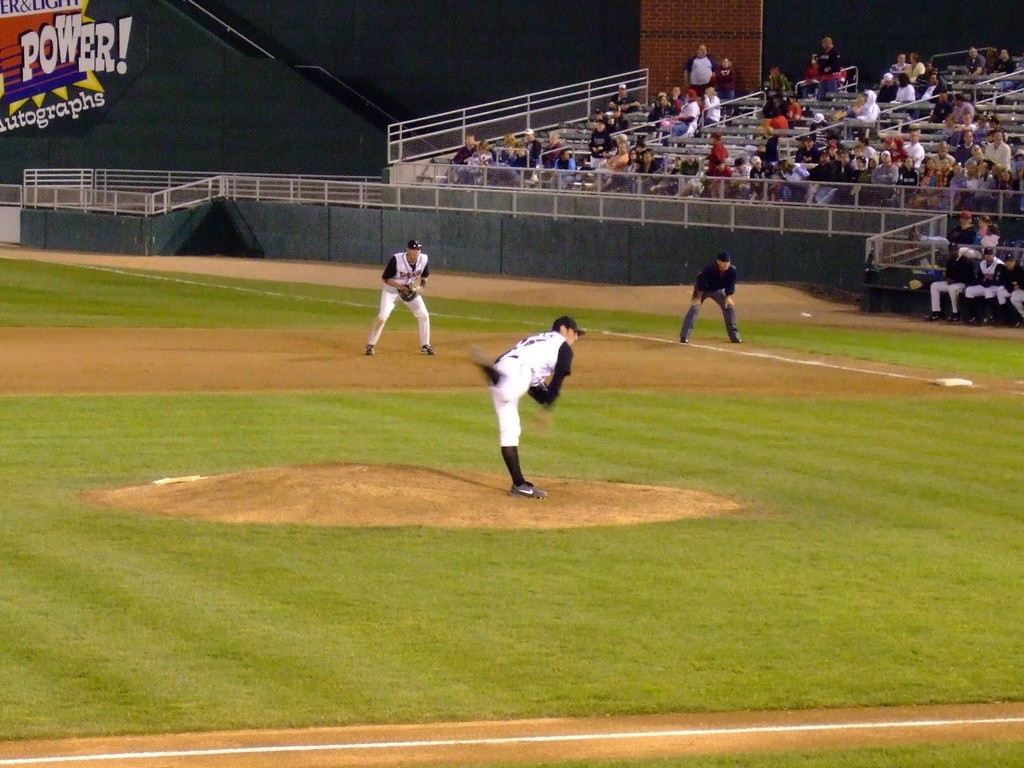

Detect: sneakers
[510, 479, 547, 500]
[365, 346, 374, 355]
[422, 344, 434, 356]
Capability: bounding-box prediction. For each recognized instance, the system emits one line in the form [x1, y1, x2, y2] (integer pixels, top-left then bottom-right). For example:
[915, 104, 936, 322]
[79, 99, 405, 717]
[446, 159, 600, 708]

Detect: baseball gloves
[397, 283, 418, 302]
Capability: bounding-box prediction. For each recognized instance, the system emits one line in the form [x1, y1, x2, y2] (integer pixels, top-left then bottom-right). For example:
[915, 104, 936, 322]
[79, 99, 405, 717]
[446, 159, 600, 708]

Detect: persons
[449, 35, 1024, 261]
[966, 247, 1006, 326]
[680, 254, 744, 344]
[997, 251, 1024, 327]
[465, 317, 582, 500]
[364, 240, 437, 356]
[928, 242, 978, 323]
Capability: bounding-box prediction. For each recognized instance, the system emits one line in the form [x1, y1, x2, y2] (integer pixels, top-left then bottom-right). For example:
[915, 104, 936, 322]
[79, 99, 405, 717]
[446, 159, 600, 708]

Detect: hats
[1003, 253, 1016, 262]
[407, 238, 421, 251]
[947, 242, 960, 253]
[982, 248, 995, 255]
[551, 312, 586, 339]
[716, 249, 732, 262]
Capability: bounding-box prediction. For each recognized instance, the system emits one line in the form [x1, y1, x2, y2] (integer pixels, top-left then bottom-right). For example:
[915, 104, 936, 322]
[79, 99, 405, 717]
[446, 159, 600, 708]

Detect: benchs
[422, 54, 1023, 324]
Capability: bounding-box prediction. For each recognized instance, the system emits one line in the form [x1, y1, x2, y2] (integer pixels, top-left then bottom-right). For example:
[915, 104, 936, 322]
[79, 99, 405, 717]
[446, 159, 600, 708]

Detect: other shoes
[732, 337, 743, 344]
[930, 309, 1024, 328]
[678, 335, 689, 343]
